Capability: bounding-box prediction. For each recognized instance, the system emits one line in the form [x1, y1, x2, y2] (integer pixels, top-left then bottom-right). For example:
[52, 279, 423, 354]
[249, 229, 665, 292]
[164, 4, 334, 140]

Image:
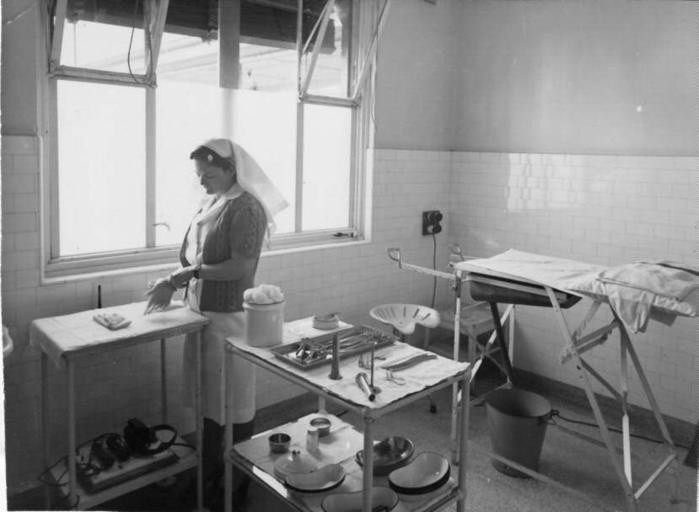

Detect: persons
[144, 139, 289, 511]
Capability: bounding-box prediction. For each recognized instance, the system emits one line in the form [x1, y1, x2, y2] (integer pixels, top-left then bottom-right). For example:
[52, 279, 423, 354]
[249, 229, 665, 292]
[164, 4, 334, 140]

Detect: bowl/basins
[285, 435, 450, 512]
[269, 432, 290, 453]
[310, 417, 330, 437]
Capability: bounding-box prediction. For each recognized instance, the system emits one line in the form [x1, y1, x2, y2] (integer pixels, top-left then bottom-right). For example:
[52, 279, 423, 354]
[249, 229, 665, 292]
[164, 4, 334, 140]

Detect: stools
[369, 303, 441, 413]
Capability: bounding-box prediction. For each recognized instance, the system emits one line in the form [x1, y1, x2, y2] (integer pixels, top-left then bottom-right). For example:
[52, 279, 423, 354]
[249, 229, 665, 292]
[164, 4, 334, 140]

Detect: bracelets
[194, 263, 201, 279]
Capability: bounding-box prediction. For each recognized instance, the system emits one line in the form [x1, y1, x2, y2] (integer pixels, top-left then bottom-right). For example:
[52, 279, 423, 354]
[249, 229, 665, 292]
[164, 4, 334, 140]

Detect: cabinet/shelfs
[224, 312, 471, 512]
[424, 302, 509, 395]
[31, 298, 211, 511]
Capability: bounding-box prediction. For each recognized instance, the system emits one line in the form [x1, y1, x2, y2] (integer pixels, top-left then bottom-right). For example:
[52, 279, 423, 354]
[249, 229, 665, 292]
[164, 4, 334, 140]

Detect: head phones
[92, 431, 131, 470]
[123, 417, 177, 456]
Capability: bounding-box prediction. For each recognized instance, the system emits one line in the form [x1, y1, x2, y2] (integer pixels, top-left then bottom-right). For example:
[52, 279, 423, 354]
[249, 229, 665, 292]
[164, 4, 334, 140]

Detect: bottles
[307, 430, 319, 453]
